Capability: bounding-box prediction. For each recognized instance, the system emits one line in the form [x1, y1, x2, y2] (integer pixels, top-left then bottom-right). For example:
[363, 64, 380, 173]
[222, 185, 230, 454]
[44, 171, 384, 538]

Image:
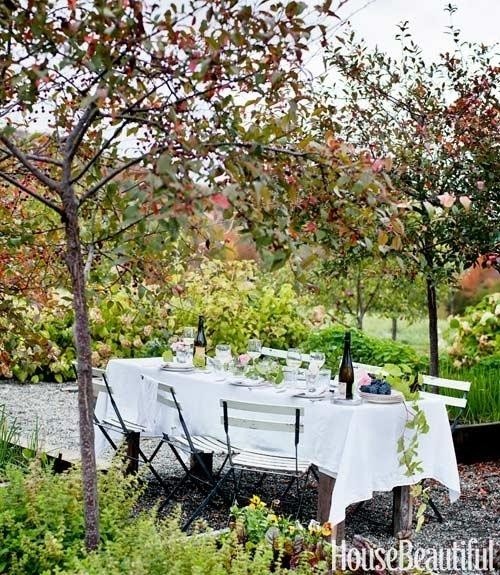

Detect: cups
[281, 347, 332, 394]
[210, 345, 231, 381]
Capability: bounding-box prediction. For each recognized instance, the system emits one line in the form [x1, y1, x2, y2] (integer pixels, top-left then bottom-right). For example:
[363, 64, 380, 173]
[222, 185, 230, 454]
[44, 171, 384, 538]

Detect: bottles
[193, 316, 207, 367]
[339, 333, 354, 398]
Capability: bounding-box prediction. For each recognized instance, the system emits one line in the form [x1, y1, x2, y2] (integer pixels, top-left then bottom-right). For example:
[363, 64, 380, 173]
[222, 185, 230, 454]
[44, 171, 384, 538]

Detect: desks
[103, 357, 445, 551]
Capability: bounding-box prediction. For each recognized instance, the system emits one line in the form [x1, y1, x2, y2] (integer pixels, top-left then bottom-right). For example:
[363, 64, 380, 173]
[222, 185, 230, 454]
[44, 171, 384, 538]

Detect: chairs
[72, 346, 472, 523]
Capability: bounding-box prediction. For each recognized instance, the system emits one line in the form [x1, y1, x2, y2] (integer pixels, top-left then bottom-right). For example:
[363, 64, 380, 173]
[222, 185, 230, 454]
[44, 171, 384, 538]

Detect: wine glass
[247, 339, 261, 366]
[181, 326, 196, 362]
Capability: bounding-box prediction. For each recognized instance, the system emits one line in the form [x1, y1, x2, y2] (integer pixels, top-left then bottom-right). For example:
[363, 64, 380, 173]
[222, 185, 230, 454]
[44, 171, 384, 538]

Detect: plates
[357, 391, 404, 405]
[160, 363, 195, 372]
[230, 379, 260, 387]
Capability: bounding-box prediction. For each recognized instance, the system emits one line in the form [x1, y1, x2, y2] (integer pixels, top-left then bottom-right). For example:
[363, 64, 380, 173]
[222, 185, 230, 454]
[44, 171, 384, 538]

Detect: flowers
[171, 341, 190, 352]
